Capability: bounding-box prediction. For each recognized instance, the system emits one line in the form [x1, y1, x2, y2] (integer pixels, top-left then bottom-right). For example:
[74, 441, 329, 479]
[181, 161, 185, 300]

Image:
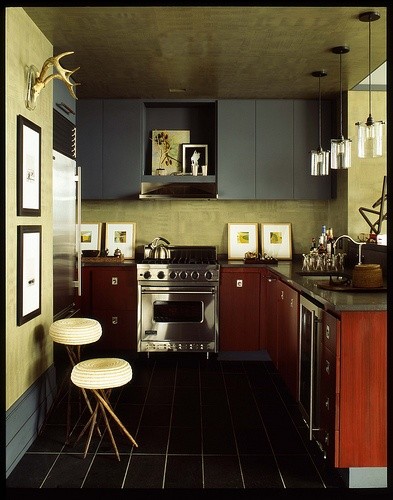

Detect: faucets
[332, 234, 366, 266]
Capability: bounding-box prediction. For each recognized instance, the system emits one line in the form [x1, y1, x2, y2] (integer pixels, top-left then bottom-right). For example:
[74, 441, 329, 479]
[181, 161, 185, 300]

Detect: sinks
[297, 271, 351, 282]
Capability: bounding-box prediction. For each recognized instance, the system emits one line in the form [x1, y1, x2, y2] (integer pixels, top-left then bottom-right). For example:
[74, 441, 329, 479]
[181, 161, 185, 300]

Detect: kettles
[148, 237, 171, 259]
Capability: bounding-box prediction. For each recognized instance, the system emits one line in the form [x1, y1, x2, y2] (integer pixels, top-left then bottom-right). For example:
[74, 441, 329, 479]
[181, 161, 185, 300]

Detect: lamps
[309, 10, 387, 177]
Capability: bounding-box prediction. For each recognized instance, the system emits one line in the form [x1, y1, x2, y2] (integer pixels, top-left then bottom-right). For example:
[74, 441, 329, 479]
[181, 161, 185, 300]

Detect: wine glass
[301, 253, 345, 272]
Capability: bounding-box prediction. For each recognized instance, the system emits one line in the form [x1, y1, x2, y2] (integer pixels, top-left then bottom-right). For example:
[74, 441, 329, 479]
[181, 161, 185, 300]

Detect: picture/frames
[77, 220, 137, 261]
[228, 222, 293, 262]
[17, 113, 42, 218]
[182, 142, 211, 176]
[16, 224, 42, 327]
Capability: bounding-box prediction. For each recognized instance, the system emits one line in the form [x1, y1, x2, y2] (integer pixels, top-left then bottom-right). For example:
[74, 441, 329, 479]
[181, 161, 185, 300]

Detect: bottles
[114, 249, 121, 258]
[309, 225, 336, 254]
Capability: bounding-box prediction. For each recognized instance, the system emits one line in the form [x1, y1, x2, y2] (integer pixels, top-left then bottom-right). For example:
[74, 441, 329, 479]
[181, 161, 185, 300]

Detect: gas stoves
[137, 244, 219, 271]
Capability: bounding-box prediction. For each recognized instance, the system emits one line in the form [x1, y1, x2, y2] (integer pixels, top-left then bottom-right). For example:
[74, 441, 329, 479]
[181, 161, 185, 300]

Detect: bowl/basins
[82, 250, 99, 257]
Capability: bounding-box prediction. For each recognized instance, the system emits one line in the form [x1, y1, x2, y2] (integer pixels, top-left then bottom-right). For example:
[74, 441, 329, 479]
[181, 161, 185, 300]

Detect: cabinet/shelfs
[77, 95, 338, 199]
[75, 267, 387, 468]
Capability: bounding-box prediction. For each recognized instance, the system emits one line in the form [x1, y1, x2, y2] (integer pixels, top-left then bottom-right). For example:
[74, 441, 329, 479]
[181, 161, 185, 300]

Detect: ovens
[137, 270, 219, 360]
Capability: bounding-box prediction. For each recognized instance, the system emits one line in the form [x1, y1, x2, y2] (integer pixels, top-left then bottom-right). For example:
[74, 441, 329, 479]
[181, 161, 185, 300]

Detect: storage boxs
[348, 242, 387, 278]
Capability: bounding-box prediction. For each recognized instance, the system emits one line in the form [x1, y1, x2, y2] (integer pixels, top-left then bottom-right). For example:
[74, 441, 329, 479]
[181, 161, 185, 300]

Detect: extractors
[139, 182, 216, 201]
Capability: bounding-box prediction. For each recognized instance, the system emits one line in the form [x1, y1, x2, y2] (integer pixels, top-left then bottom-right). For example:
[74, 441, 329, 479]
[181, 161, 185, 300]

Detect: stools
[48, 318, 139, 462]
